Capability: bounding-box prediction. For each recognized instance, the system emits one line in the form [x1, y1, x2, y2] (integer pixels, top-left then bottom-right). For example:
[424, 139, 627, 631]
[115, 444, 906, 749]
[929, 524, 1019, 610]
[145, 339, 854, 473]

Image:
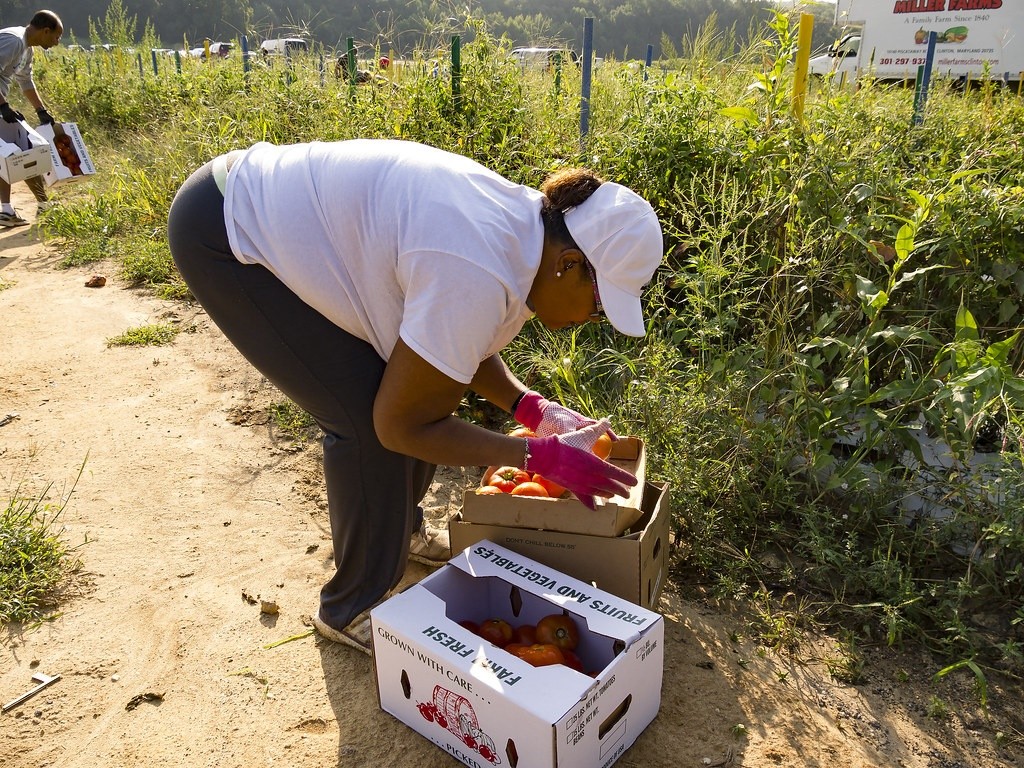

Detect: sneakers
[35, 207, 46, 218]
[409, 515, 451, 568]
[313, 586, 392, 657]
[0, 208, 30, 226]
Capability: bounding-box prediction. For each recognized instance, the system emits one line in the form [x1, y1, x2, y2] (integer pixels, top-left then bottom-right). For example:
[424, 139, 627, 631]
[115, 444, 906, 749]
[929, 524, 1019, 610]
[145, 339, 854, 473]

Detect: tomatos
[52, 135, 83, 176]
[454, 615, 600, 679]
[476, 428, 612, 499]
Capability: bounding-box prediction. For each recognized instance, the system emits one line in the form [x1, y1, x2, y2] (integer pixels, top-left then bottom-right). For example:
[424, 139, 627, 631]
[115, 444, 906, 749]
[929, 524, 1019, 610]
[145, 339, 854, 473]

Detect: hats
[561, 181, 664, 338]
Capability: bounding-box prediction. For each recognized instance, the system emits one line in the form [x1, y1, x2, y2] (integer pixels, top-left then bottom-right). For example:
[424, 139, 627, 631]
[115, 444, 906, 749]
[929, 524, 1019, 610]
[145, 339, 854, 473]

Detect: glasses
[583, 257, 606, 317]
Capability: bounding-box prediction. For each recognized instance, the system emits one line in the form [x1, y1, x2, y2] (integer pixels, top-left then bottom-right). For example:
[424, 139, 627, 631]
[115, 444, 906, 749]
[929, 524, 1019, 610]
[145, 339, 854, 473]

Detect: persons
[0, 7, 64, 230]
[164, 134, 664, 661]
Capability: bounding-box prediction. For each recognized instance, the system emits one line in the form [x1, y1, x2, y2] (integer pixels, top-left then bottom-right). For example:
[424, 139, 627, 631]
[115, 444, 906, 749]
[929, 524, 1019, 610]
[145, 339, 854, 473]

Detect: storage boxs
[461, 436, 647, 537]
[0, 115, 52, 185]
[447, 482, 670, 612]
[35, 122, 97, 188]
[368, 539, 665, 768]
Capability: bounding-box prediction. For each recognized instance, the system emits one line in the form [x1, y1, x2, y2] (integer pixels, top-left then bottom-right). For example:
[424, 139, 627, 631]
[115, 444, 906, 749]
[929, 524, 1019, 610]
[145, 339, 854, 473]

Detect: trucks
[806, 0, 1024, 96]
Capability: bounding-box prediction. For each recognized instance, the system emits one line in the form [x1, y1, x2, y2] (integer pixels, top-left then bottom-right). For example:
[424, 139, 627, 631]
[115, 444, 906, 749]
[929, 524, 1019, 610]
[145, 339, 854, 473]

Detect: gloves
[513, 390, 622, 444]
[0, 102, 22, 123]
[518, 417, 638, 511]
[37, 110, 55, 126]
